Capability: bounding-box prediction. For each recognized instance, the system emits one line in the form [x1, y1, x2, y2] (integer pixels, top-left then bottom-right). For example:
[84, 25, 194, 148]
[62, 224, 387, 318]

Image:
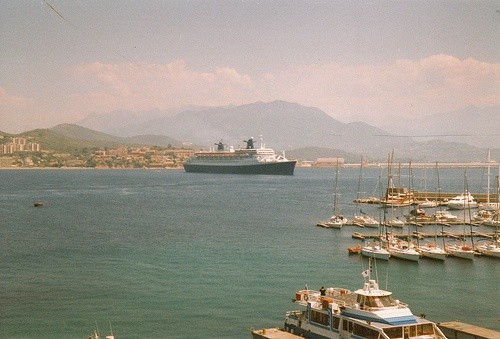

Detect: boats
[282, 252, 448, 339]
[182, 136, 297, 176]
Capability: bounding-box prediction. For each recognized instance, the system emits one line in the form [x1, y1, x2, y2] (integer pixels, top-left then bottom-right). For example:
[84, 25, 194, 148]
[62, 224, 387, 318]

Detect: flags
[304, 285, 308, 289]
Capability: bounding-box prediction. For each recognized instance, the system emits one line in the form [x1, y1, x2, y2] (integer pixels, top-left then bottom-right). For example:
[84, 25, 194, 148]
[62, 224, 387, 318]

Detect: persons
[323, 285, 327, 298]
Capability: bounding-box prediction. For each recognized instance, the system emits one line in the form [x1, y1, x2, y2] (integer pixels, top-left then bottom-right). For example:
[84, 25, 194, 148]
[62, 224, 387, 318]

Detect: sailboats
[316, 145, 500, 264]
